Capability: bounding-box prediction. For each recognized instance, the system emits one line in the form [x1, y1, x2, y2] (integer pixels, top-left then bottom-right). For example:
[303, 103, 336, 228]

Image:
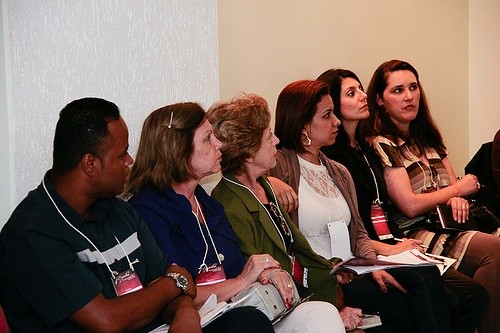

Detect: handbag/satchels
[230, 269, 300, 321]
[425, 199, 500, 233]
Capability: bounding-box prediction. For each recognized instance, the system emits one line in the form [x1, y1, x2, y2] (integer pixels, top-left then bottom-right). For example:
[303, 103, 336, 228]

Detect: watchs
[166, 272, 189, 296]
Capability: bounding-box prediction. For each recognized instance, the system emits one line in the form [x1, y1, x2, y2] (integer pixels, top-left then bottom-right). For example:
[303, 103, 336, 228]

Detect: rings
[477, 181, 481, 190]
[288, 284, 292, 289]
[265, 257, 268, 262]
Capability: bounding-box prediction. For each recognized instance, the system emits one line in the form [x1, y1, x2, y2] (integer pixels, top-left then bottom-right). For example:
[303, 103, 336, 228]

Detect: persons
[0, 60, 500, 333]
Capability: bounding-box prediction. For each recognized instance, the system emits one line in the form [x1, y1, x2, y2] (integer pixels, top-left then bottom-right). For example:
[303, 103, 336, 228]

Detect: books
[436, 204, 477, 231]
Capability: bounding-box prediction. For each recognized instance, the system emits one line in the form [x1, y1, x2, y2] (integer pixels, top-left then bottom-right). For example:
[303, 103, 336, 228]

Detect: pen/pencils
[456, 177, 487, 187]
[394, 238, 429, 248]
[358, 315, 375, 317]
